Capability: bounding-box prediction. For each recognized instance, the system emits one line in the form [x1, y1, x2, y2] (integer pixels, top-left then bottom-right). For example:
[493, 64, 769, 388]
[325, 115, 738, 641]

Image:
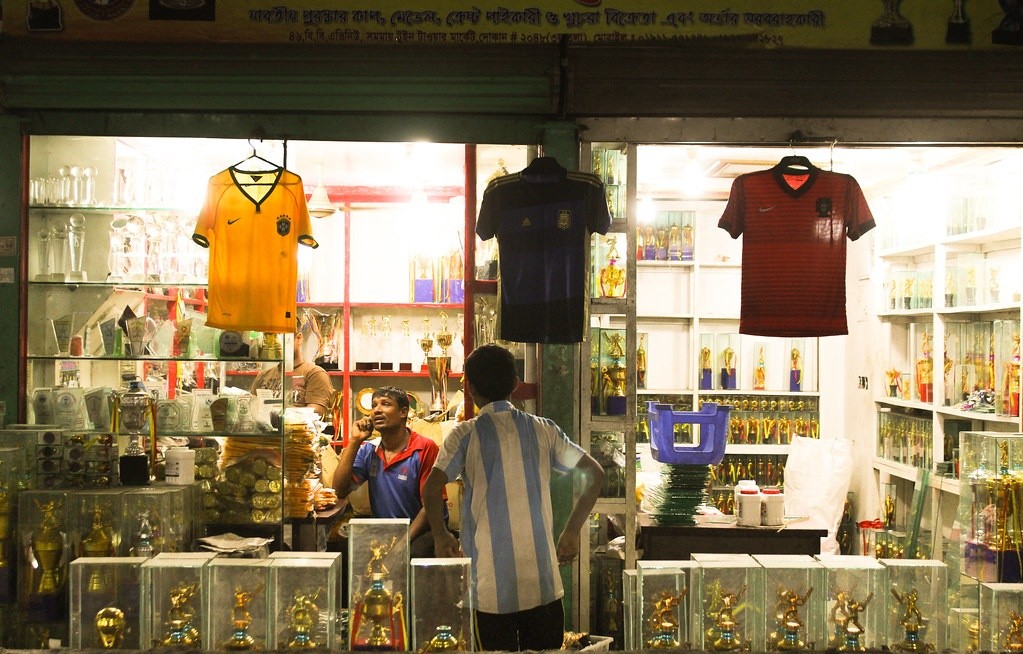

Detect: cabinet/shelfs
[28, 202, 1023, 624]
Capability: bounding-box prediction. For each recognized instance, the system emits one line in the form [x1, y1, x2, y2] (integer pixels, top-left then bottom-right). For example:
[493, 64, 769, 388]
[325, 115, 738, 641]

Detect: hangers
[209, 135, 301, 185]
[520, 133, 565, 180]
[777, 140, 812, 175]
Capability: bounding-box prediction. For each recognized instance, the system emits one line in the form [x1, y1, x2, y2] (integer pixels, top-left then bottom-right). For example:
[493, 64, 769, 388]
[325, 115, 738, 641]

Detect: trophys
[0, 148, 1023, 654]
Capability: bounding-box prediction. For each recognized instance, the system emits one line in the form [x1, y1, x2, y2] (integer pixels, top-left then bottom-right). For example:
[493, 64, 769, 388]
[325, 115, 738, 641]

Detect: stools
[644, 400, 734, 464]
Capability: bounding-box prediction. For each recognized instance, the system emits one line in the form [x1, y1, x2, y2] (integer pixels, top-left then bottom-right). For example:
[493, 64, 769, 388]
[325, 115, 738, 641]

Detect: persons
[332, 386, 450, 572]
[423, 344, 605, 652]
[250, 316, 332, 420]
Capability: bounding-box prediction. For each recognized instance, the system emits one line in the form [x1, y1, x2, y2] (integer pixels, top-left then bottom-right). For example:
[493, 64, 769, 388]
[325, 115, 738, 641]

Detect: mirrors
[356, 388, 376, 415]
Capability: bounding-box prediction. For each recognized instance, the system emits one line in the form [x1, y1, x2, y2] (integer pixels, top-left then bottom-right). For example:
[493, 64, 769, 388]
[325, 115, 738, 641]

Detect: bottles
[734, 480, 760, 518]
[165, 446, 195, 485]
[761, 488, 784, 526]
[737, 490, 761, 527]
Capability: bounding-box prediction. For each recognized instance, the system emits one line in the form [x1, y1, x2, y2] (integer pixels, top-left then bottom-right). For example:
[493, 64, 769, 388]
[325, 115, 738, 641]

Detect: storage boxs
[945, 264, 961, 308]
[917, 270, 934, 307]
[954, 321, 991, 405]
[637, 560, 702, 651]
[698, 562, 767, 652]
[622, 569, 688, 651]
[959, 432, 1023, 582]
[813, 554, 878, 646]
[818, 561, 886, 652]
[980, 583, 1023, 654]
[950, 607, 979, 653]
[879, 558, 948, 654]
[758, 562, 827, 652]
[993, 319, 1020, 418]
[751, 554, 815, 650]
[910, 321, 934, 404]
[690, 553, 754, 649]
[0, 422, 476, 654]
[898, 270, 918, 310]
